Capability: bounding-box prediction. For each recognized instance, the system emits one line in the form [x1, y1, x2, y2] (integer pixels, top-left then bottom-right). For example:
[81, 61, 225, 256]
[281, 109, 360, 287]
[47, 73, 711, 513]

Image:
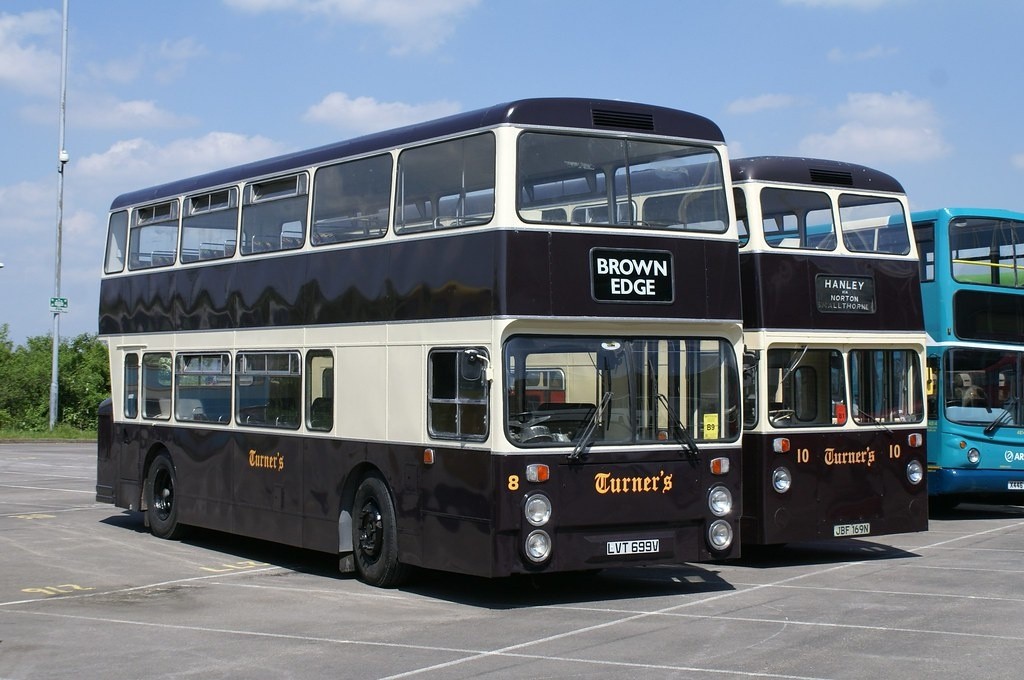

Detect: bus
[96, 97, 744, 588]
[324, 156, 929, 545]
[738, 206, 1024, 496]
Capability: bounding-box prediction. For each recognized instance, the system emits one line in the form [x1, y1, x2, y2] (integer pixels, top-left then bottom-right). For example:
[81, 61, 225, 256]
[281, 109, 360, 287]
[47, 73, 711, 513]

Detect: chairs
[158, 392, 330, 430]
[125, 207, 491, 261]
[536, 402, 601, 439]
